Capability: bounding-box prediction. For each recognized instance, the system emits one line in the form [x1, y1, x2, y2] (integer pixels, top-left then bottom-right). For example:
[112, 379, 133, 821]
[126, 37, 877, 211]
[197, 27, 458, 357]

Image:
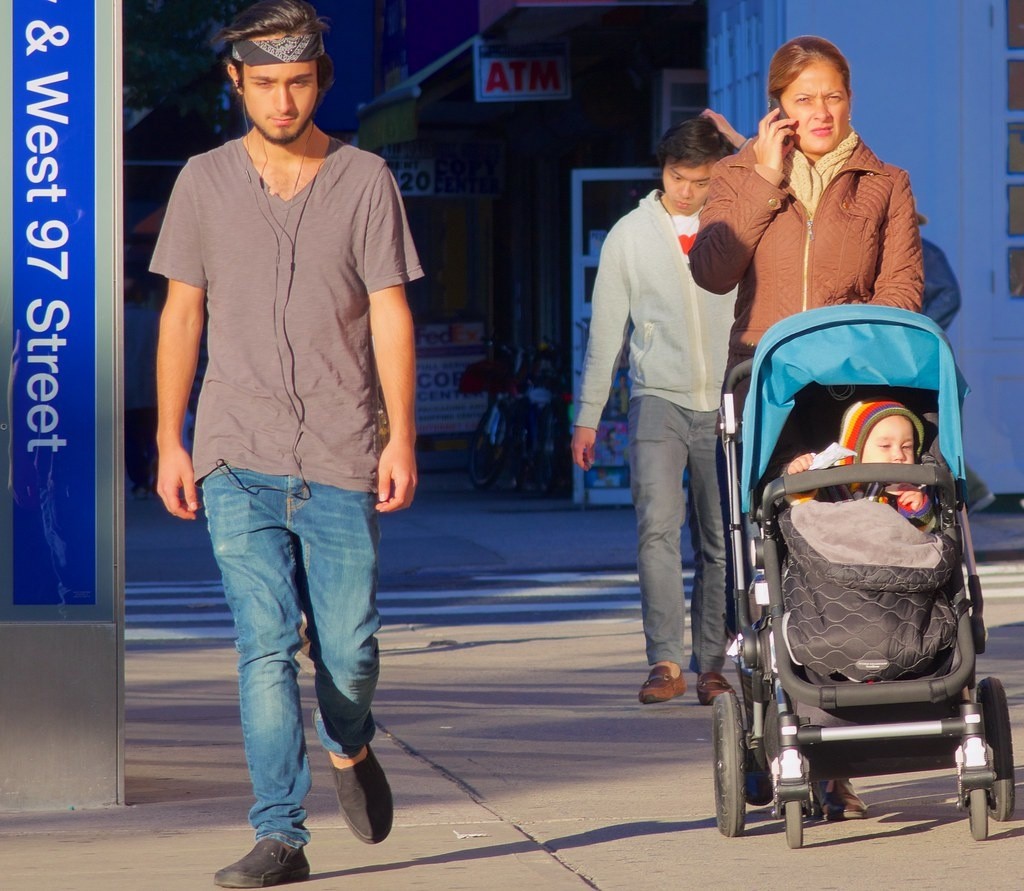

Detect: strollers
[711, 304, 1015, 851]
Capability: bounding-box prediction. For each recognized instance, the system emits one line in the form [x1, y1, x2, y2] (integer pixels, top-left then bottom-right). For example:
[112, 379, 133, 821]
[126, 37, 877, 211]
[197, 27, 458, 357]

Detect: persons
[687, 35, 926, 820]
[149, 0, 426, 889]
[571, 106, 754, 705]
[775, 398, 939, 533]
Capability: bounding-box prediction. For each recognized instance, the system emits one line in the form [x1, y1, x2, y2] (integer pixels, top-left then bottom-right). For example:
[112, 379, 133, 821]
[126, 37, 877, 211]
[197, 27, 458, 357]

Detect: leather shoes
[745, 750, 773, 805]
[812, 778, 868, 820]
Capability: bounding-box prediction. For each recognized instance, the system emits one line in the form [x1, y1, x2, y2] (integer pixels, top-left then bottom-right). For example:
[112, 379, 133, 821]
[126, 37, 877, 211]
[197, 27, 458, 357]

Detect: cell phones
[768, 95, 792, 144]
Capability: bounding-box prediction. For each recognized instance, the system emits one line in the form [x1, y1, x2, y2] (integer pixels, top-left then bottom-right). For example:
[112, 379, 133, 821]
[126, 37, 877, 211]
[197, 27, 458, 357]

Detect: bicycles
[469, 332, 570, 498]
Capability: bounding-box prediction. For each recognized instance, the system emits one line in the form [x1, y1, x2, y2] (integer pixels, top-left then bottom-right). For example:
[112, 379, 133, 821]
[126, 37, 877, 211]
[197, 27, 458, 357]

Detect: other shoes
[214, 838, 310, 888]
[639, 665, 687, 704]
[697, 671, 736, 705]
[311, 705, 393, 844]
